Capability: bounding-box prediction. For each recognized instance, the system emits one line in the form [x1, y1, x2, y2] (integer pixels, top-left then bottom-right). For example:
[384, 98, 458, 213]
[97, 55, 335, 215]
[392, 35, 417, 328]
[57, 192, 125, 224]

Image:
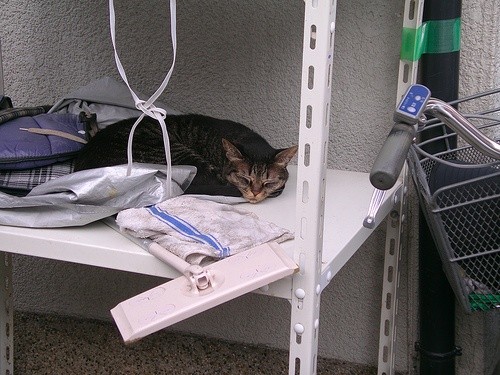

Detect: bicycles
[371, 87, 500, 315]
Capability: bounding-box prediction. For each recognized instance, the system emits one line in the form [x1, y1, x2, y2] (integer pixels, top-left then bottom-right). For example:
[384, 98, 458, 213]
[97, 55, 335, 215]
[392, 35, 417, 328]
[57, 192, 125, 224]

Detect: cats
[72, 113, 299, 204]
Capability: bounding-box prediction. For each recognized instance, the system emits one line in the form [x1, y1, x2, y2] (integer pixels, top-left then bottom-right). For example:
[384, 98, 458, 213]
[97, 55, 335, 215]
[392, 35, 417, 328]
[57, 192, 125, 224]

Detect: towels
[115, 193, 295, 266]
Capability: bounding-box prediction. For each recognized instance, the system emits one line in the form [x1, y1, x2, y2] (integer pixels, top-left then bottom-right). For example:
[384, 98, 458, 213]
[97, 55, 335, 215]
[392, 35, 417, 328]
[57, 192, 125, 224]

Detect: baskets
[409, 90, 500, 315]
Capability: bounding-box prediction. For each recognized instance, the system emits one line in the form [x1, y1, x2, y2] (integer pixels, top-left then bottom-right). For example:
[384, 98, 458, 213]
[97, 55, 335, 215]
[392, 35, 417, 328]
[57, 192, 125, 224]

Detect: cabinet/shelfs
[1, 0, 425, 375]
